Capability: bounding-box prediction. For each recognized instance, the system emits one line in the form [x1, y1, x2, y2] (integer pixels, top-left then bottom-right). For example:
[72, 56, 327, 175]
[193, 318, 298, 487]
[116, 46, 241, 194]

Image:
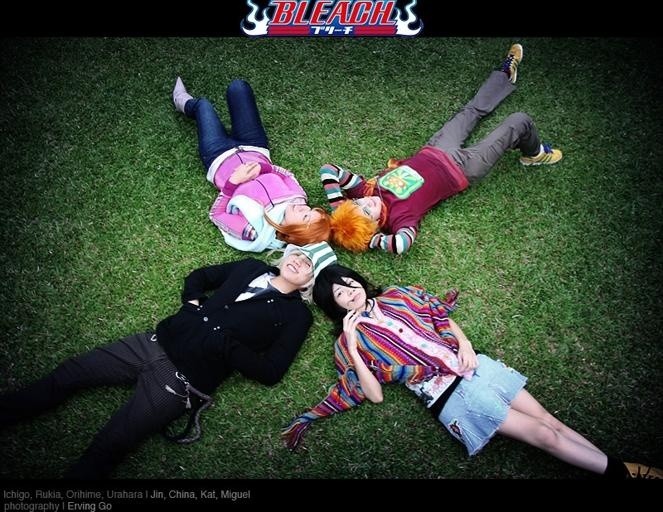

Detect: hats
[285, 241, 338, 286]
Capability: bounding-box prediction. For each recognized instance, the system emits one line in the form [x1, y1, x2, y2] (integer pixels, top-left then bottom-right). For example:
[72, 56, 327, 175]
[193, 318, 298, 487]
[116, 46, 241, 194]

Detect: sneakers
[622, 460, 661, 482]
[519, 145, 563, 165]
[506, 43, 524, 84]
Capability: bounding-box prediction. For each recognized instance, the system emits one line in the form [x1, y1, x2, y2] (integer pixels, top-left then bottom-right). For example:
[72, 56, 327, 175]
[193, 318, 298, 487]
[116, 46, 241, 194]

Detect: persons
[0, 240, 338, 480]
[318, 42, 564, 256]
[312, 263, 663, 479]
[172, 75, 333, 255]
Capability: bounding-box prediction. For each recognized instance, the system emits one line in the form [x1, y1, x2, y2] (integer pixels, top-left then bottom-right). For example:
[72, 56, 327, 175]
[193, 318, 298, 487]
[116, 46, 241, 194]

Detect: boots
[173, 76, 193, 113]
